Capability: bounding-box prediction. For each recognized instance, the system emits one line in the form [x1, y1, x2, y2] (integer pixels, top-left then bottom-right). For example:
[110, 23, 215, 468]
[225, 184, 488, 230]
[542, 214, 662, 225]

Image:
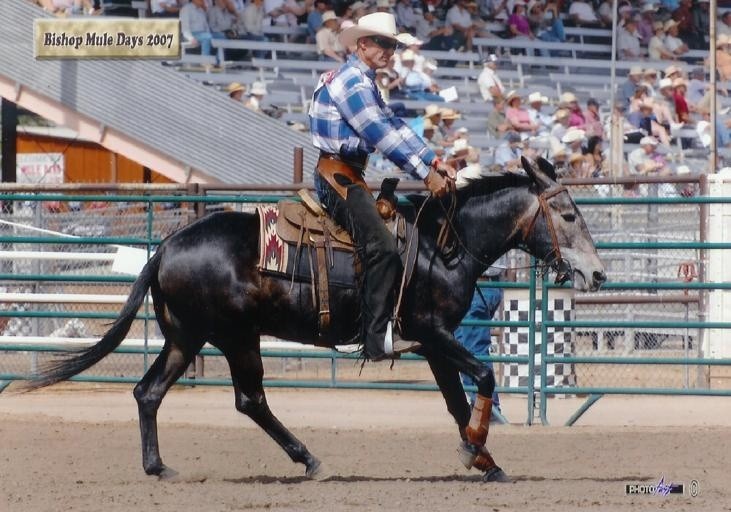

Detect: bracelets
[434, 159, 442, 170]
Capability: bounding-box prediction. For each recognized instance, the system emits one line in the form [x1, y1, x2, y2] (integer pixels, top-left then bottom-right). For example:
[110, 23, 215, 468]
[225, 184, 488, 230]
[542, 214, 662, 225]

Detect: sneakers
[366, 336, 420, 359]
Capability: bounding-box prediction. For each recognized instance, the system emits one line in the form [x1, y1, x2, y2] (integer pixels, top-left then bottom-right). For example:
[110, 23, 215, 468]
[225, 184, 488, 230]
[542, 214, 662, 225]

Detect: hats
[508, 134, 523, 144]
[249, 81, 269, 96]
[618, 3, 693, 175]
[562, 92, 576, 104]
[562, 129, 584, 144]
[321, 0, 473, 152]
[513, 0, 537, 16]
[553, 108, 570, 125]
[504, 89, 522, 107]
[226, 81, 245, 97]
[567, 152, 588, 166]
[527, 92, 548, 105]
[587, 98, 602, 105]
[485, 53, 503, 65]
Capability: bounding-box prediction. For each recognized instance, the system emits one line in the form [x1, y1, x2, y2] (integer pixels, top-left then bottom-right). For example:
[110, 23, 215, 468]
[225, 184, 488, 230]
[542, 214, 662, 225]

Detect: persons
[28, 1, 731, 198]
[307, 11, 458, 364]
[453, 164, 510, 424]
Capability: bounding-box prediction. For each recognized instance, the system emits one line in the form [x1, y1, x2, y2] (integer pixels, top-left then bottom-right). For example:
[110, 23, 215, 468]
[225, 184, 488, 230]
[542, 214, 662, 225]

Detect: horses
[11, 154, 609, 483]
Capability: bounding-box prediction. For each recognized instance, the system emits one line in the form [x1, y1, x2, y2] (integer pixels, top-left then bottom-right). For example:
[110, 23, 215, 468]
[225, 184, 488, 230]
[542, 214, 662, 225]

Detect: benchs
[39, 1, 730, 210]
